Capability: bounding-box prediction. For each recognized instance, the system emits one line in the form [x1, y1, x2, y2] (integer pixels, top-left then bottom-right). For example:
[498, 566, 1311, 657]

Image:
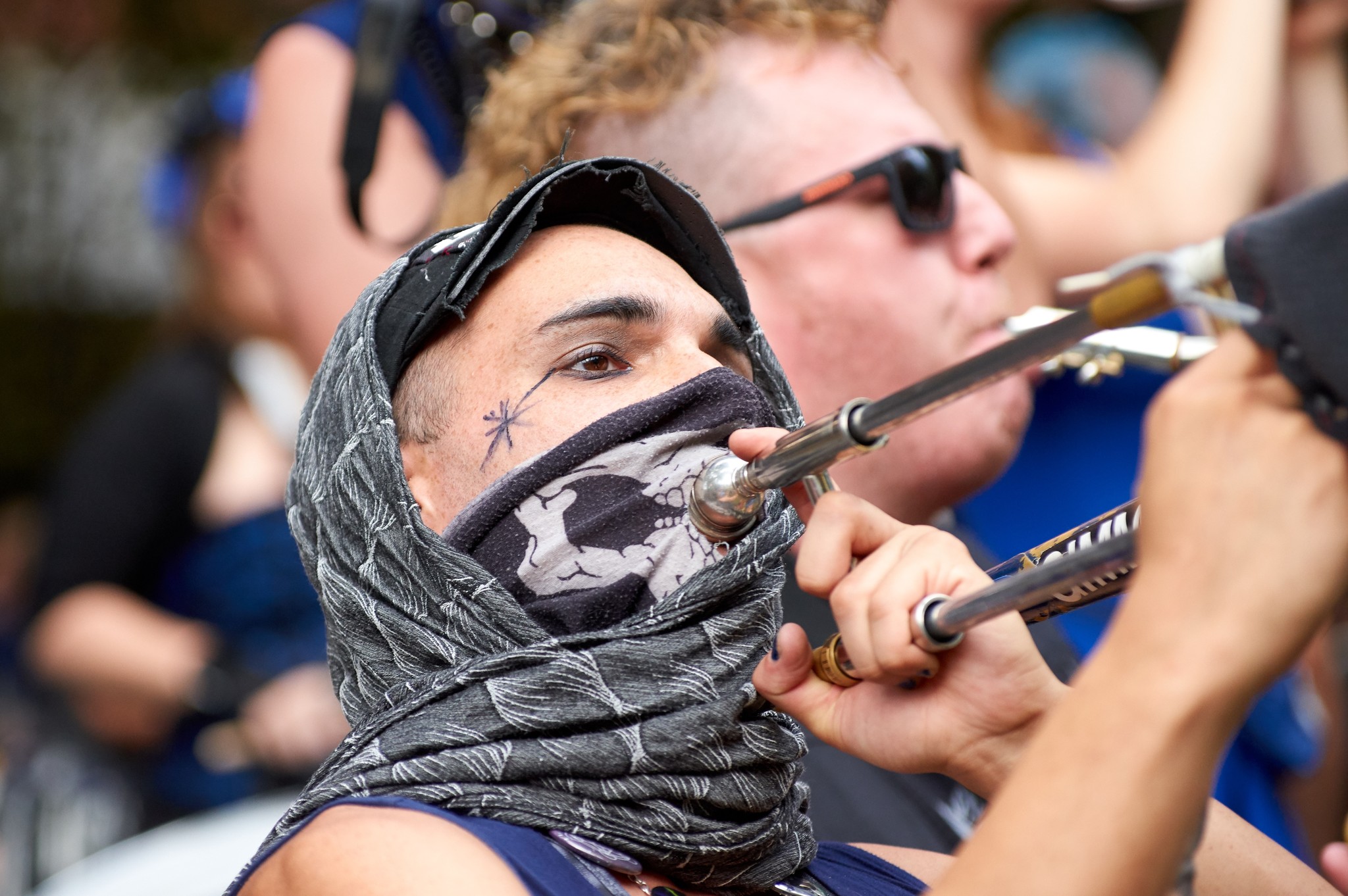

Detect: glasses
[720, 141, 965, 243]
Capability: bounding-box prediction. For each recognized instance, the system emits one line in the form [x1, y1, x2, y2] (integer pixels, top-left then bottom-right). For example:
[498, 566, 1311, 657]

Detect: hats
[375, 152, 758, 393]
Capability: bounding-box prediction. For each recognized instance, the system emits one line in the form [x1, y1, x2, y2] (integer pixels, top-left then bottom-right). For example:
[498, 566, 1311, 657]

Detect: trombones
[677, 173, 1348, 691]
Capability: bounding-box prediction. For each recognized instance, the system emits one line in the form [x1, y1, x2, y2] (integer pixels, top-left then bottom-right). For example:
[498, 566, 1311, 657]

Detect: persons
[424, 0, 1055, 546]
[146, 0, 486, 837]
[872, 0, 1295, 292]
[8, 70, 267, 839]
[215, 143, 1346, 896]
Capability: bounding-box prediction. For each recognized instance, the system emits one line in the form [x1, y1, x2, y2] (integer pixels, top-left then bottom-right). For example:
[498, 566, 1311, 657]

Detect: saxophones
[990, 242, 1227, 376]
